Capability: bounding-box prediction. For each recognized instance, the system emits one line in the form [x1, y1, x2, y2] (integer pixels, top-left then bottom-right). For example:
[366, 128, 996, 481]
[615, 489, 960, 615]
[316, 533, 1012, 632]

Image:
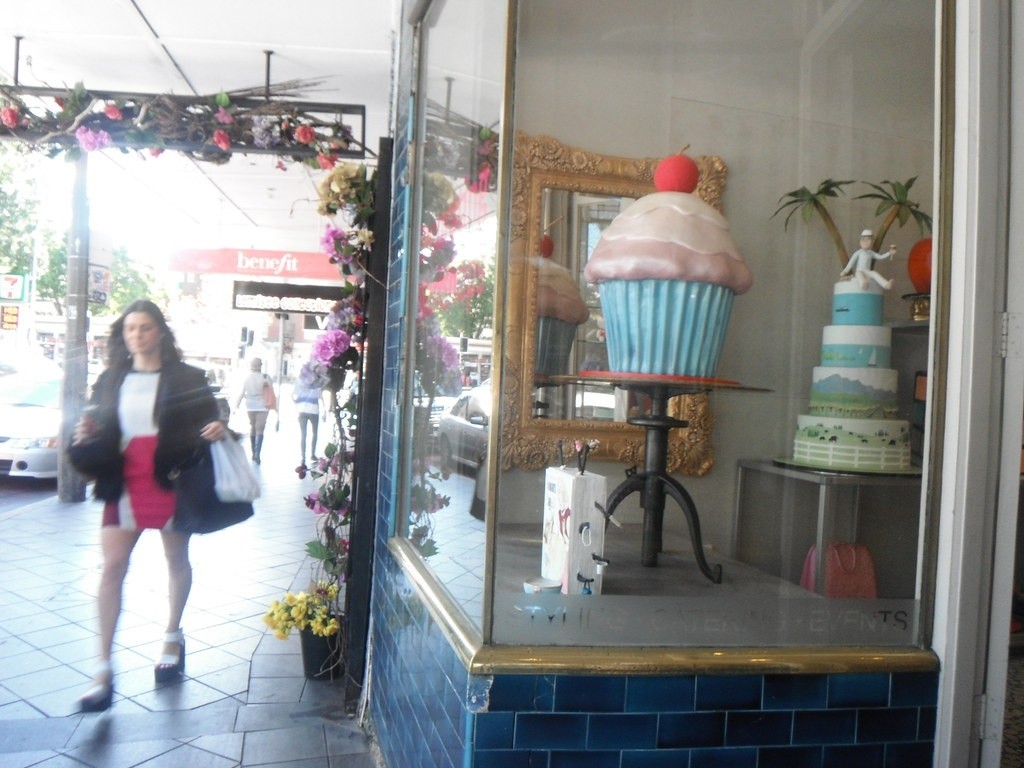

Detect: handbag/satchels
[173, 430, 256, 535]
[262, 374, 276, 409]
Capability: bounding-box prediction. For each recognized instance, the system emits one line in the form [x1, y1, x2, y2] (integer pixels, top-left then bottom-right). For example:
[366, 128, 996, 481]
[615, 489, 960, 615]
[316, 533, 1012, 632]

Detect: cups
[80, 404, 102, 443]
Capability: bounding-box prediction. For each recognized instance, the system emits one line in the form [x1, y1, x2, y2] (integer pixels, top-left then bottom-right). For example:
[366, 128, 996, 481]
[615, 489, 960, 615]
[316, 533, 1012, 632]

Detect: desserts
[583, 144, 754, 380]
[535, 235, 589, 378]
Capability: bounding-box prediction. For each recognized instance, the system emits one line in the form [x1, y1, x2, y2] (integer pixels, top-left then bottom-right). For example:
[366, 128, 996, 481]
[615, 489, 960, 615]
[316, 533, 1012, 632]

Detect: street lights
[276, 311, 288, 409]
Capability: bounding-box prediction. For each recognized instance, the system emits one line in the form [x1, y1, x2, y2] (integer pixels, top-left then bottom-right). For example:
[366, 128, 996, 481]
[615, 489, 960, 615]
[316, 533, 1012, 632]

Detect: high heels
[81, 659, 113, 713]
[153, 627, 185, 682]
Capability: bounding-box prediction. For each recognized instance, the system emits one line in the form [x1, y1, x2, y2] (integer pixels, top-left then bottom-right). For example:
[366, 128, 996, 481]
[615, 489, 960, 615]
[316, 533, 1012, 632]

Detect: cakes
[791, 227, 913, 473]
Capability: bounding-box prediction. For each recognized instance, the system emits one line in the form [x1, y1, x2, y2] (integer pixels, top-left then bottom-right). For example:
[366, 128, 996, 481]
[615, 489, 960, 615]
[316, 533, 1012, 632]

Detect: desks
[729, 445, 924, 601]
[539, 369, 776, 591]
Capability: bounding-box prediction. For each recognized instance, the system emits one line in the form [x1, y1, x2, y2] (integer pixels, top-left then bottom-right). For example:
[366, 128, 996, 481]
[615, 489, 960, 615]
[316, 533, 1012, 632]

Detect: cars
[1, 350, 93, 409]
[435, 384, 493, 476]
[575, 390, 616, 422]
[182, 358, 231, 431]
[0, 375, 100, 482]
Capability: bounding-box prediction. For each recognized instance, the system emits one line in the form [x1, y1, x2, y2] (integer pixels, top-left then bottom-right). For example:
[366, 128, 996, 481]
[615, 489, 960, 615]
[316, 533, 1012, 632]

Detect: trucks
[414, 370, 460, 453]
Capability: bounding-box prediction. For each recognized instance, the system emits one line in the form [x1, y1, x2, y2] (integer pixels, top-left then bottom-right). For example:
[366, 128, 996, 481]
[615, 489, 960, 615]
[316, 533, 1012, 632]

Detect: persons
[235, 358, 279, 467]
[73, 297, 226, 714]
[293, 362, 325, 467]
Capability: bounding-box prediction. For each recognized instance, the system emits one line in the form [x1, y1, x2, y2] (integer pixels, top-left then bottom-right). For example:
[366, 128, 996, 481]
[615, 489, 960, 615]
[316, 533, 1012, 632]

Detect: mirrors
[496, 127, 729, 481]
